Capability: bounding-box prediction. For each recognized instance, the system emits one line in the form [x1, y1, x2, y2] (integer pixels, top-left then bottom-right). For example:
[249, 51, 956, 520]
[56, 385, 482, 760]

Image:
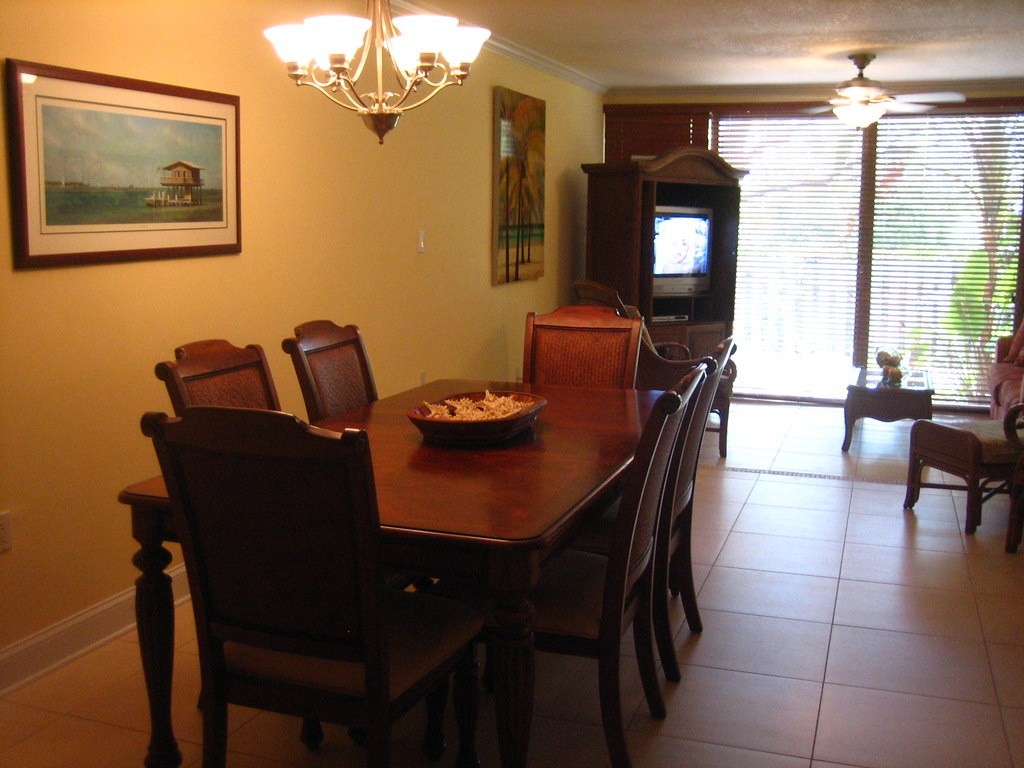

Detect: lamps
[262, 0, 491, 145]
[828, 96, 896, 131]
[837, 54, 885, 101]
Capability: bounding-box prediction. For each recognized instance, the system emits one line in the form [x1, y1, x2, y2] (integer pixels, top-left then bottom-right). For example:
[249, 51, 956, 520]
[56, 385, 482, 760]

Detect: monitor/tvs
[654, 205, 715, 297]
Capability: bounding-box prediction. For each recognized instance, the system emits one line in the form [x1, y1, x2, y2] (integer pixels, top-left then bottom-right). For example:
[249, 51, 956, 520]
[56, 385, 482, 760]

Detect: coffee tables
[841, 366, 936, 453]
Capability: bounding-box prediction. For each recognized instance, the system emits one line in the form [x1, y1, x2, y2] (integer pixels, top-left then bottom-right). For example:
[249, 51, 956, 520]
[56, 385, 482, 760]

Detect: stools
[903, 417, 1024, 537]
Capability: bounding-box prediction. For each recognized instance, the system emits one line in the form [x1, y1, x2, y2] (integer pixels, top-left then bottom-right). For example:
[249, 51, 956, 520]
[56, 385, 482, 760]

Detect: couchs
[987, 335, 1024, 421]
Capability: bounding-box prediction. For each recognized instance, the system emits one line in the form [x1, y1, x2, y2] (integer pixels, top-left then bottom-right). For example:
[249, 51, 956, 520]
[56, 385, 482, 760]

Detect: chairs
[466, 361, 709, 768]
[573, 278, 736, 458]
[522, 304, 645, 389]
[155, 340, 283, 414]
[575, 335, 737, 687]
[282, 321, 379, 425]
[140, 405, 483, 768]
[1003, 402, 1024, 555]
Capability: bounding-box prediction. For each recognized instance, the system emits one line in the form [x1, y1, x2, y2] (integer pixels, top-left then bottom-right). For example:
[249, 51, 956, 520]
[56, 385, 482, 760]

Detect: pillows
[1003, 318, 1024, 362]
[1011, 345, 1024, 366]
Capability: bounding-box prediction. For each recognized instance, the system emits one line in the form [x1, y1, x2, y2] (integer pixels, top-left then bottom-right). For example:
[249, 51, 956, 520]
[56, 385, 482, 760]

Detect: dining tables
[116, 377, 674, 767]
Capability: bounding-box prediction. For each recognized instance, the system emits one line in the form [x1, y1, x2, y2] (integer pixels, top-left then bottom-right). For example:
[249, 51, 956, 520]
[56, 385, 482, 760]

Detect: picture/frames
[5, 57, 242, 271]
[491, 85, 546, 288]
